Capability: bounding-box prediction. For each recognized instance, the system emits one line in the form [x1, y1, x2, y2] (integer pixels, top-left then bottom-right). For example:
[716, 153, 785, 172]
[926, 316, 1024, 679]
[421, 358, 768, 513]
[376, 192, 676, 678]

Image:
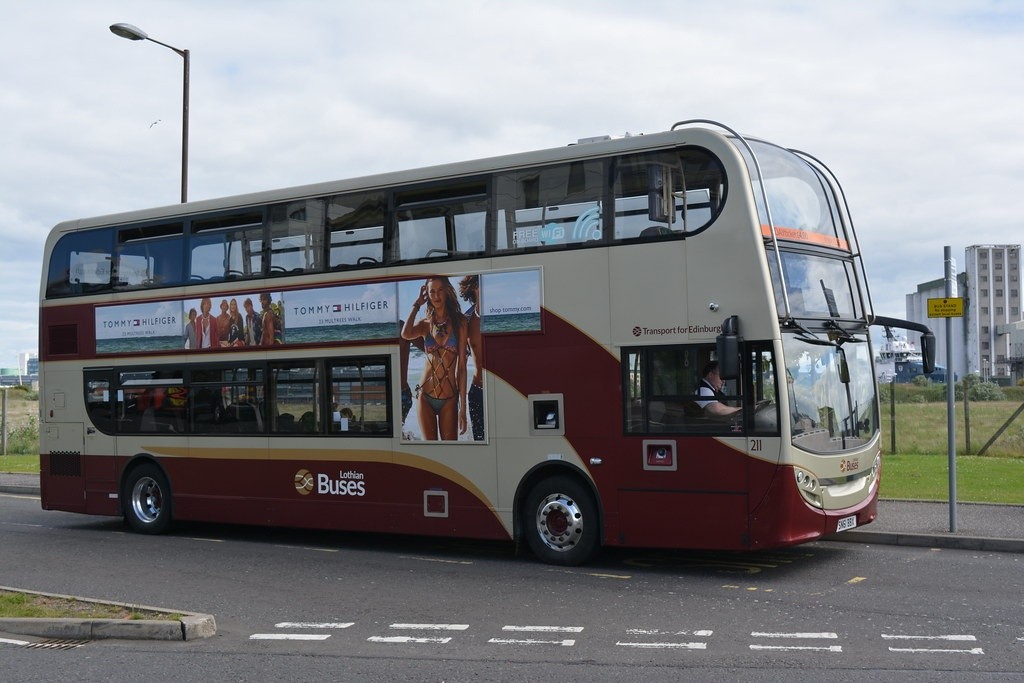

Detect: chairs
[85, 227, 696, 438]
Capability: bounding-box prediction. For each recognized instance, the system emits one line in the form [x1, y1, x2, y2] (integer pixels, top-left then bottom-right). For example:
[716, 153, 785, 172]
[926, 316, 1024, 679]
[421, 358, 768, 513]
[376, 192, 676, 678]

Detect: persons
[339, 407, 354, 423]
[259, 293, 285, 345]
[242, 297, 262, 346]
[685, 361, 742, 418]
[227, 299, 244, 347]
[184, 308, 198, 349]
[459, 274, 484, 441]
[216, 300, 231, 349]
[195, 298, 220, 350]
[399, 320, 425, 426]
[401, 275, 469, 440]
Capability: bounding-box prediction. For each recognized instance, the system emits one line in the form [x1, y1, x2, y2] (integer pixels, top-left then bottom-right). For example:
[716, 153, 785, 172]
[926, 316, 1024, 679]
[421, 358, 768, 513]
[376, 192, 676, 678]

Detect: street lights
[109, 19, 195, 203]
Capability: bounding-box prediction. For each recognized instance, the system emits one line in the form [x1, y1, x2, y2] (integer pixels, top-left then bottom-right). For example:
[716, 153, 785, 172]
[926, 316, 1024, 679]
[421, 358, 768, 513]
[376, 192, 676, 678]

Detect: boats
[876, 323, 957, 384]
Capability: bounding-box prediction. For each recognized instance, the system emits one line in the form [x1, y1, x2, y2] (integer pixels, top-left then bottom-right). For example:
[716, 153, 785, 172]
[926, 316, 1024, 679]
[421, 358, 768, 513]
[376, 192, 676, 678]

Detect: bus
[34, 120, 941, 572]
